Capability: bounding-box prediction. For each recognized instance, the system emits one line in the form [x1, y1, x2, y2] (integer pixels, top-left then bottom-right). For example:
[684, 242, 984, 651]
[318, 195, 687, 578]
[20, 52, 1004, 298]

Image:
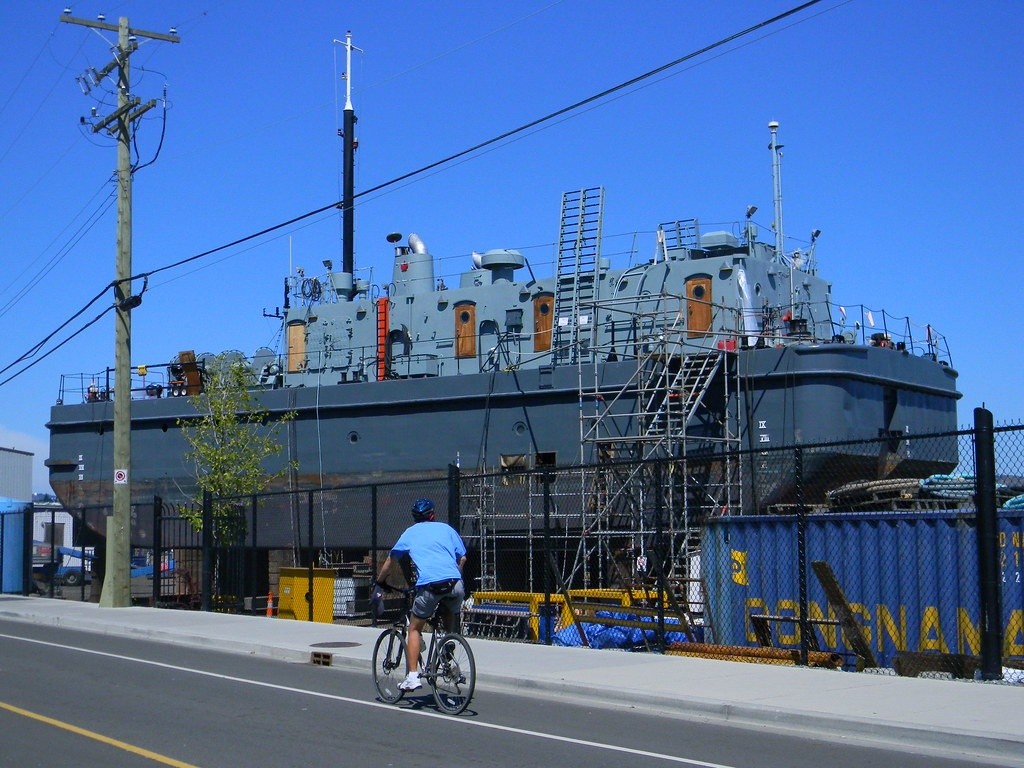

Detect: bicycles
[370, 577, 478, 715]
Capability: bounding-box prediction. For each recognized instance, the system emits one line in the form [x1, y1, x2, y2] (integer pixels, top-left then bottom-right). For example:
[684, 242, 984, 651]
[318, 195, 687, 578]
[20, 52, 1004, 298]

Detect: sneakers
[447, 660, 461, 676]
[396, 675, 422, 690]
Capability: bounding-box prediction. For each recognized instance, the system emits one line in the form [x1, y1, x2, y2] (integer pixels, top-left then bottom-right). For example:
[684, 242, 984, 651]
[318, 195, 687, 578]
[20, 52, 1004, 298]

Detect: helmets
[411, 497, 435, 521]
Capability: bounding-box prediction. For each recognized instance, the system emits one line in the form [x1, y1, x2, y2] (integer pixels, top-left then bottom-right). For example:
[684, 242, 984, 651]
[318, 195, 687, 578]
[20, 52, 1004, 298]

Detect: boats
[43, 28, 958, 590]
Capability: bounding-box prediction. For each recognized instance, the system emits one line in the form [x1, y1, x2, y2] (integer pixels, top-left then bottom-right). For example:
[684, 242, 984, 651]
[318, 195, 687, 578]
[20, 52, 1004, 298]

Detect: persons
[375, 498, 468, 692]
